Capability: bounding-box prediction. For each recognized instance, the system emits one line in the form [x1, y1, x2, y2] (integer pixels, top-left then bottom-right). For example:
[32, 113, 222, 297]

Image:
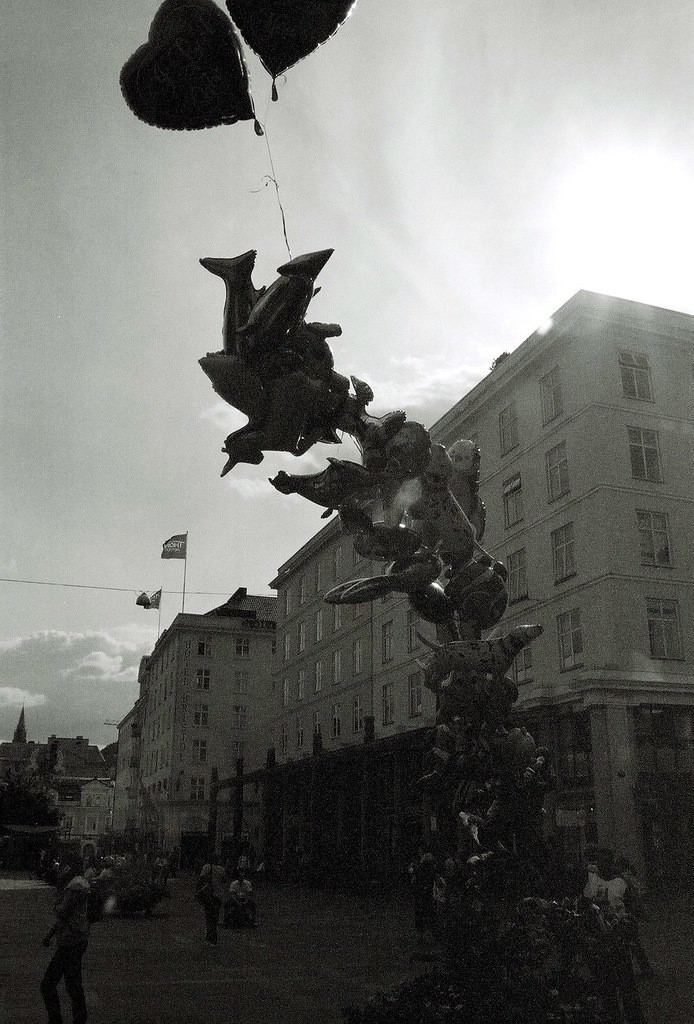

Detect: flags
[160, 533, 187, 559]
[144, 588, 161, 610]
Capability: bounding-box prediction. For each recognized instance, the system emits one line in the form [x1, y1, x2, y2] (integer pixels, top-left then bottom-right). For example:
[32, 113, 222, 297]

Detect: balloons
[197, 249, 626, 981]
[121, 0, 264, 139]
[227, 0, 351, 101]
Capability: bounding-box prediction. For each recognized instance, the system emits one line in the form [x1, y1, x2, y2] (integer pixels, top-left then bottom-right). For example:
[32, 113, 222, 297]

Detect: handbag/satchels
[194, 864, 215, 905]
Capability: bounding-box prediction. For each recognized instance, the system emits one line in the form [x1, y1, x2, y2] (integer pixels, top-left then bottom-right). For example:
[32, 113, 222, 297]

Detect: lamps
[135, 592, 152, 606]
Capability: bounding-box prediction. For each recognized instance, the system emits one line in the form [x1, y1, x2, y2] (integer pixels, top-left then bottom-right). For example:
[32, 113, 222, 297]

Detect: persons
[199, 853, 227, 948]
[41, 851, 90, 1024]
[236, 848, 250, 877]
[576, 845, 654, 1024]
[228, 871, 253, 906]
